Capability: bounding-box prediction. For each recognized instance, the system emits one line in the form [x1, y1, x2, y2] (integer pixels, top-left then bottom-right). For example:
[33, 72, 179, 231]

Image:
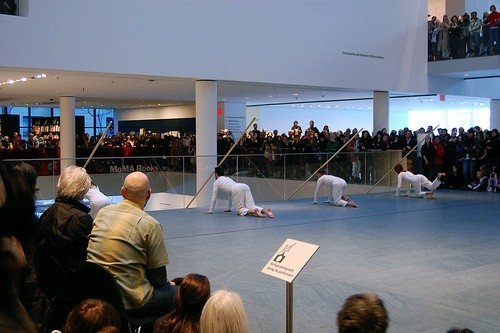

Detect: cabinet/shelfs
[32, 115, 85, 143]
[0, 113, 20, 141]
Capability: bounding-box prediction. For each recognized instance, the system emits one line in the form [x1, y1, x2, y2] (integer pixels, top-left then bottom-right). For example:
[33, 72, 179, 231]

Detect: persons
[313, 170, 359, 208]
[63, 298, 121, 333]
[200, 291, 247, 333]
[87, 171, 180, 323]
[0, 161, 39, 333]
[427, 5, 500, 62]
[34, 166, 93, 300]
[395, 164, 445, 200]
[152, 274, 209, 333]
[0, 120, 500, 192]
[207, 167, 273, 218]
[336, 294, 388, 333]
